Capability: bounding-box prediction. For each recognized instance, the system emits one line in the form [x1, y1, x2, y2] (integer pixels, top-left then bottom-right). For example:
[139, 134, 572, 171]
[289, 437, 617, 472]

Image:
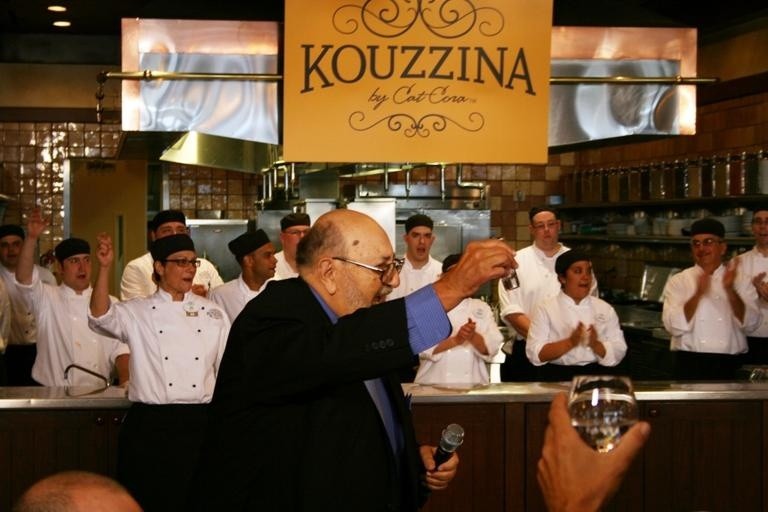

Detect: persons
[93, 233, 232, 504]
[388, 213, 442, 302]
[272, 212, 309, 280]
[119, 209, 223, 299]
[415, 253, 504, 386]
[499, 206, 599, 378]
[1, 221, 57, 387]
[16, 473, 144, 512]
[533, 392, 651, 512]
[193, 208, 518, 512]
[662, 218, 763, 379]
[12, 202, 129, 393]
[729, 210, 766, 360]
[525, 248, 628, 382]
[205, 230, 278, 321]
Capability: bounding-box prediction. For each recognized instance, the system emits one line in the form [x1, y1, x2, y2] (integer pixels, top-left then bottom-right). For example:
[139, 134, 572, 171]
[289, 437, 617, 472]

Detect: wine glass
[567, 375, 639, 452]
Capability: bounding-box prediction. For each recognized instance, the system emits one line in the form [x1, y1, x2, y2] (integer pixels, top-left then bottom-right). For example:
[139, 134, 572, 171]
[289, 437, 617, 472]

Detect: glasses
[532, 219, 559, 230]
[690, 238, 723, 247]
[163, 259, 201, 269]
[332, 256, 405, 284]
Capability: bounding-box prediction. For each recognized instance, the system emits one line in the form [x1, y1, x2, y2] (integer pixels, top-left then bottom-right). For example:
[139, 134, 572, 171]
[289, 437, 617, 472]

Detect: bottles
[494, 235, 523, 290]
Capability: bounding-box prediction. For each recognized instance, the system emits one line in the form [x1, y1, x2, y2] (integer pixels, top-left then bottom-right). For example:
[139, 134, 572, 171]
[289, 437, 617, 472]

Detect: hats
[753, 203, 768, 215]
[280, 213, 310, 231]
[150, 210, 184, 233]
[443, 254, 461, 273]
[555, 246, 593, 275]
[405, 215, 433, 234]
[529, 206, 559, 221]
[681, 218, 725, 240]
[150, 234, 194, 262]
[55, 237, 90, 263]
[228, 229, 268, 258]
[1, 224, 25, 243]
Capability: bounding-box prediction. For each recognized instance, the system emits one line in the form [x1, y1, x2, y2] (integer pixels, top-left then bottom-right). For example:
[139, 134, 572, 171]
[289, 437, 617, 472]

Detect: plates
[741, 211, 756, 236]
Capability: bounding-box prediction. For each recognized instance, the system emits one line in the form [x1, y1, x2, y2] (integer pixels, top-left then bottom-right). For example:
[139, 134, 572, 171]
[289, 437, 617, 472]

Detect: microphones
[422, 424, 465, 467]
